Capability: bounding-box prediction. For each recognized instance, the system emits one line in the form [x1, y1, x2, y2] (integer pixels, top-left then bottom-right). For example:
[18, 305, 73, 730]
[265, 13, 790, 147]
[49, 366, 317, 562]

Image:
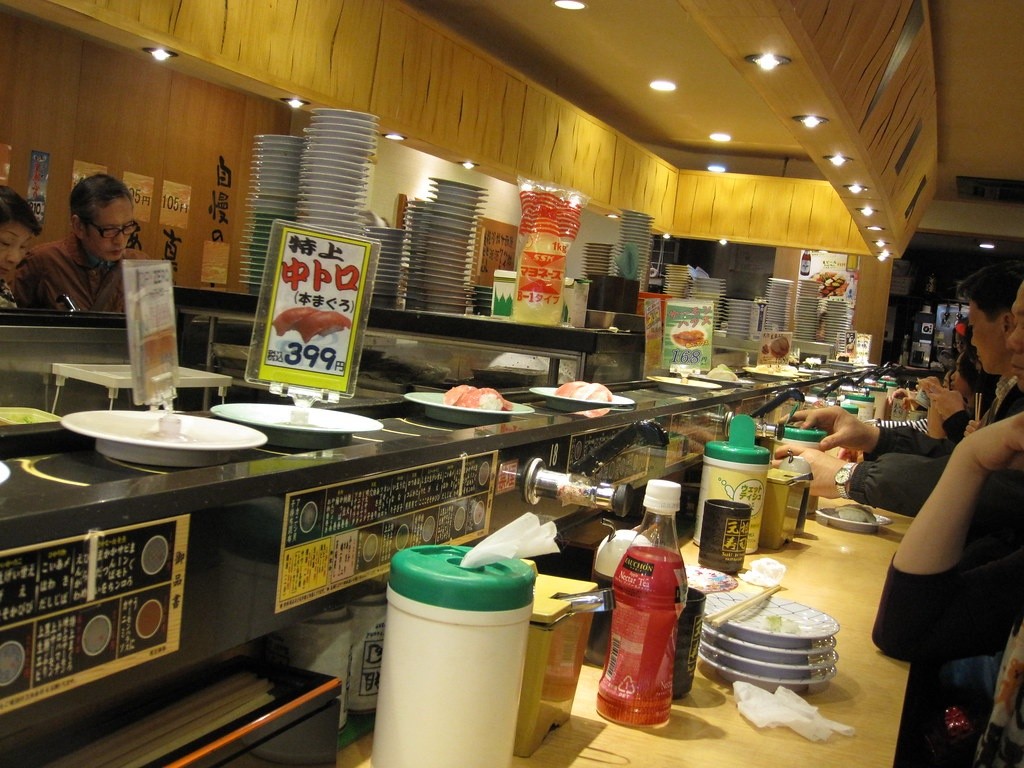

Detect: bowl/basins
[585, 310, 615, 329]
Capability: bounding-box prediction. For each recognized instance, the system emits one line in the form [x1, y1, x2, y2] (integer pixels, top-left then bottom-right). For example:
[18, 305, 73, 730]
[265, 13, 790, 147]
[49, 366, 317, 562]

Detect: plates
[404, 392, 535, 425]
[663, 264, 756, 340]
[529, 387, 634, 412]
[690, 375, 755, 387]
[61, 411, 268, 467]
[646, 376, 722, 395]
[765, 278, 851, 353]
[581, 209, 655, 292]
[210, 403, 384, 449]
[698, 592, 841, 693]
[814, 508, 894, 533]
[742, 367, 799, 382]
[685, 566, 739, 592]
[799, 367, 830, 374]
[238, 108, 492, 316]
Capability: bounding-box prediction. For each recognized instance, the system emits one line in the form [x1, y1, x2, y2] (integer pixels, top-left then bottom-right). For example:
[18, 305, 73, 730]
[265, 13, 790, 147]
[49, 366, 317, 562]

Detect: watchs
[834, 462, 856, 500]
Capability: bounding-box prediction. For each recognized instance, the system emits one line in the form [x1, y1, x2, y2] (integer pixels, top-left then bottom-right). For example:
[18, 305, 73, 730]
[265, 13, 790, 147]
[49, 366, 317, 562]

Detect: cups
[915, 387, 930, 409]
[909, 410, 927, 421]
[698, 499, 752, 572]
[673, 588, 706, 700]
[891, 398, 906, 421]
[807, 496, 818, 514]
[272, 592, 389, 737]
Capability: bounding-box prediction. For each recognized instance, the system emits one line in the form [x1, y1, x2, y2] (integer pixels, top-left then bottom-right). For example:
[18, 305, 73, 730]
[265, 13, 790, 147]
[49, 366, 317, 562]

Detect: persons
[0, 183, 42, 311]
[773, 259, 1024, 554]
[10, 171, 152, 313]
[872, 412, 1023, 768]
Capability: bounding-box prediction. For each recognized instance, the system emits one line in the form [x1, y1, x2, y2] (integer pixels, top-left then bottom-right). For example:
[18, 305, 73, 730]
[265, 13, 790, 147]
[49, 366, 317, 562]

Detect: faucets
[815, 374, 857, 399]
[749, 386, 806, 419]
[569, 417, 670, 478]
[855, 370, 880, 385]
[874, 364, 895, 382]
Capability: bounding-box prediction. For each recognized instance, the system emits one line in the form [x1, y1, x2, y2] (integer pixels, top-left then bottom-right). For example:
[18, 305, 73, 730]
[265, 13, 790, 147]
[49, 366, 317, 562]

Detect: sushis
[711, 364, 738, 380]
[574, 383, 612, 403]
[446, 385, 476, 407]
[835, 504, 877, 522]
[557, 382, 586, 397]
[275, 308, 319, 332]
[464, 388, 513, 412]
[299, 312, 350, 341]
[813, 273, 848, 298]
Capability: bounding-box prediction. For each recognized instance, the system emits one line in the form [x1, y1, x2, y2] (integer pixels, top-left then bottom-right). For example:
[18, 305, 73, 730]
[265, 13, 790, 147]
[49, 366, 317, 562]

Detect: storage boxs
[513, 560, 601, 758]
[636, 292, 672, 324]
[584, 309, 645, 334]
[758, 468, 807, 548]
[585, 275, 641, 314]
[490, 269, 593, 328]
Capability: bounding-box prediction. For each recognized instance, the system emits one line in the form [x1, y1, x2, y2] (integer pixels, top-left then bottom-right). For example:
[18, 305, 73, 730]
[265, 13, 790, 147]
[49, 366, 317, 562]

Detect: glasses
[90, 222, 138, 238]
[952, 340, 965, 349]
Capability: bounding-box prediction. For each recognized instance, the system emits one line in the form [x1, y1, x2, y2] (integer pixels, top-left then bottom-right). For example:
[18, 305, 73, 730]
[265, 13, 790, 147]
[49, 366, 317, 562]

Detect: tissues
[375, 511, 560, 768]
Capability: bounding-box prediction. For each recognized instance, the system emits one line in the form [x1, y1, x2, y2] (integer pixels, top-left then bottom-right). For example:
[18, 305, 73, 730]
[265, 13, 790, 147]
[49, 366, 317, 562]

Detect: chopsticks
[704, 584, 781, 627]
[975, 393, 982, 421]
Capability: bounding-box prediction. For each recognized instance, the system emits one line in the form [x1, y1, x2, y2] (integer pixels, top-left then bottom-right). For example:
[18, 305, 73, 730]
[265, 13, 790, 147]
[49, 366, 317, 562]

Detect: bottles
[597, 480, 688, 730]
